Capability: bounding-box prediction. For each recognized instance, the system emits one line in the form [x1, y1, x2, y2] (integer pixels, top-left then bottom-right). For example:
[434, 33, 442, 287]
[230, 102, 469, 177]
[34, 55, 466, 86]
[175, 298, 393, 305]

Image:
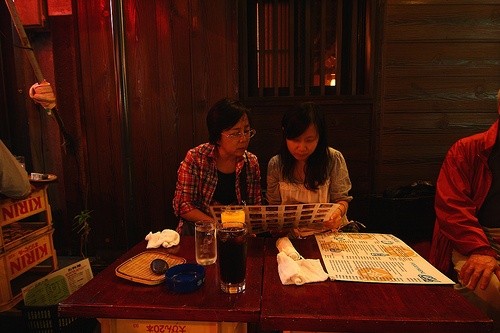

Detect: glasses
[222, 129, 256, 139]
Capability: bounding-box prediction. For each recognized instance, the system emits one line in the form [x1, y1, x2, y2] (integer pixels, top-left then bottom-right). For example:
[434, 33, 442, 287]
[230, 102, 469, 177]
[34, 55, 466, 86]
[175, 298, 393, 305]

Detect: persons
[266, 100, 354, 229]
[173, 97, 263, 236]
[429, 90, 500, 311]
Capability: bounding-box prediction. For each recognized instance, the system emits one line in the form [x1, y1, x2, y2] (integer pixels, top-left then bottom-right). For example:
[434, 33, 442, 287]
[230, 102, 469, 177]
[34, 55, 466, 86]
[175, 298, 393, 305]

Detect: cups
[222, 210, 245, 229]
[16, 156, 25, 170]
[216, 222, 249, 294]
[195, 220, 216, 266]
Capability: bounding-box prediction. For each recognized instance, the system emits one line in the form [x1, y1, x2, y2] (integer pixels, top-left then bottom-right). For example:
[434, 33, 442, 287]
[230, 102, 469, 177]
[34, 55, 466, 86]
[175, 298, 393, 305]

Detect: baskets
[15, 265, 108, 333]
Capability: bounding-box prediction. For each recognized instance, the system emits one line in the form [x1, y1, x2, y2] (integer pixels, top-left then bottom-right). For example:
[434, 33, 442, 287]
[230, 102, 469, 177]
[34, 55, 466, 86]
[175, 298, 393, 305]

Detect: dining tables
[260, 236, 495, 333]
[57, 235, 266, 333]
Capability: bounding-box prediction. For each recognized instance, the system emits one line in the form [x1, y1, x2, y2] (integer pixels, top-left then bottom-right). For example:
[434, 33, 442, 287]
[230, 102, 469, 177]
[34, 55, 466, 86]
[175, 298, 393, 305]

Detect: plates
[28, 173, 57, 187]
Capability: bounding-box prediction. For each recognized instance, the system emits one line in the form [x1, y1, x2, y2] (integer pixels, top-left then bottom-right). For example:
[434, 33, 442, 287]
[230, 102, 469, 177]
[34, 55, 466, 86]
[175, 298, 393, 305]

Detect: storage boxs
[0, 184, 60, 313]
[13, 267, 88, 333]
